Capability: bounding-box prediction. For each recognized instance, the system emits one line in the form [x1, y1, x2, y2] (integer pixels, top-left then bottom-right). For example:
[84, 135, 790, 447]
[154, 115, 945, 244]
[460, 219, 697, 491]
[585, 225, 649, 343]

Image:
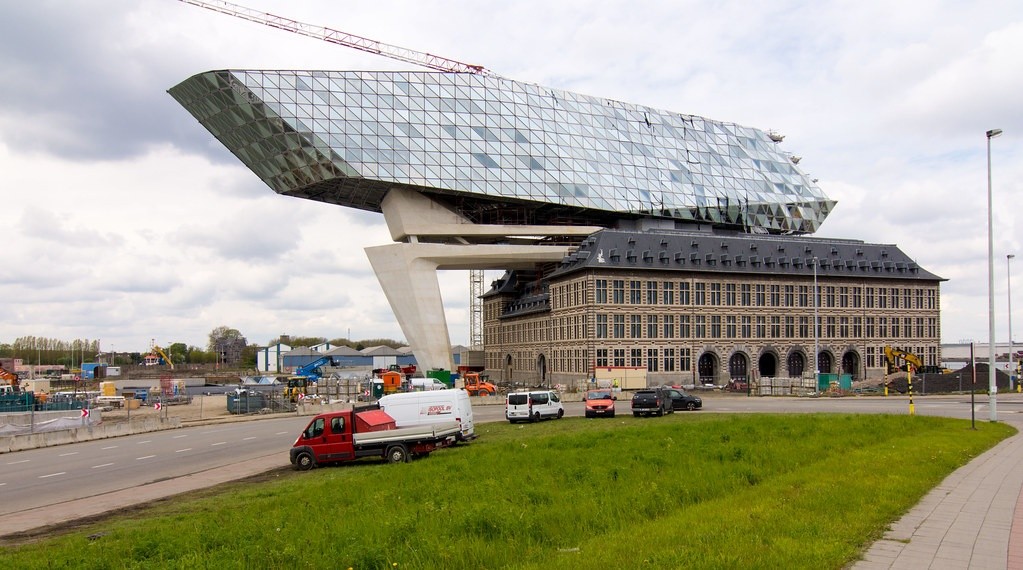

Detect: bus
[55, 391, 100, 403]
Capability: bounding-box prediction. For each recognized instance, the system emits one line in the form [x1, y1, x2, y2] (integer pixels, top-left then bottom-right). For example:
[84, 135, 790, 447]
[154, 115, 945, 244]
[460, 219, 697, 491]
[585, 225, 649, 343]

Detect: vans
[505, 391, 564, 423]
[409, 378, 447, 391]
[375, 388, 480, 447]
[96, 395, 126, 407]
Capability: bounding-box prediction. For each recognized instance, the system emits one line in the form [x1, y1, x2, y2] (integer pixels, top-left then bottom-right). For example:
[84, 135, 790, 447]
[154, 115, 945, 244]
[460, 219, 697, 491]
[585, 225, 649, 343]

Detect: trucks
[290, 401, 462, 471]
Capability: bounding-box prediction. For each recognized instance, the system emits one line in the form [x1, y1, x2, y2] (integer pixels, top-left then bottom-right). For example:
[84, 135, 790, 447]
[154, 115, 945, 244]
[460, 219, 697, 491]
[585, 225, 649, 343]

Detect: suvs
[631, 389, 673, 417]
[584, 390, 617, 417]
[663, 389, 702, 411]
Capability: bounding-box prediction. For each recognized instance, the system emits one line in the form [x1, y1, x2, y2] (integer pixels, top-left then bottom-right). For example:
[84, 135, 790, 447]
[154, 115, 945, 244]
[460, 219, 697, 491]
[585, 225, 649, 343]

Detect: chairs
[333, 422, 339, 431]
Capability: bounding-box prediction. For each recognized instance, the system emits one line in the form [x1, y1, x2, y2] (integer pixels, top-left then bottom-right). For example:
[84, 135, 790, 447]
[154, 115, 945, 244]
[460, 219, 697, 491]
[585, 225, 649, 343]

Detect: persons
[612, 378, 618, 388]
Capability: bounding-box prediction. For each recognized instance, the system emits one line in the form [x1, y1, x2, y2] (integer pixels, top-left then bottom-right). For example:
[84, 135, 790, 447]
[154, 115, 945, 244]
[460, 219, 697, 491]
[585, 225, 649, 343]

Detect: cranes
[180, 0, 484, 74]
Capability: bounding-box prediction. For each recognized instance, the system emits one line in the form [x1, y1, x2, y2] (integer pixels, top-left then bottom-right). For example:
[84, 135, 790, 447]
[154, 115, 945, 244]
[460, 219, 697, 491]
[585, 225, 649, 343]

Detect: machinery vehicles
[885, 345, 944, 374]
[463, 373, 499, 397]
[296, 354, 341, 382]
[0, 367, 49, 410]
[284, 376, 308, 403]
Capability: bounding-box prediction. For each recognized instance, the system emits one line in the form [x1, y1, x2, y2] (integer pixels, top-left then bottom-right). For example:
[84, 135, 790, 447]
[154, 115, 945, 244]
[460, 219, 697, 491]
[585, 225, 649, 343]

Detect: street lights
[220, 344, 224, 368]
[986, 128, 1003, 423]
[812, 257, 819, 398]
[1007, 255, 1014, 391]
